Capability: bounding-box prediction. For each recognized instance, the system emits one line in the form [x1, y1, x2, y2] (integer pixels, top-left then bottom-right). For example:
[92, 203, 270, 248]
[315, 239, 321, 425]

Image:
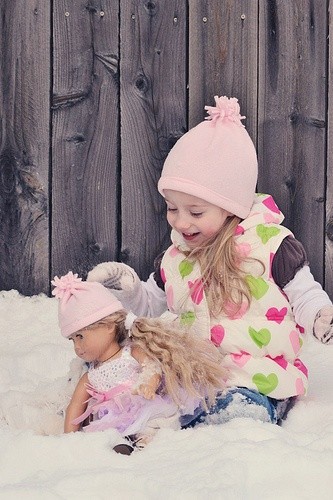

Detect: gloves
[86, 261, 134, 294]
[314, 305, 332, 344]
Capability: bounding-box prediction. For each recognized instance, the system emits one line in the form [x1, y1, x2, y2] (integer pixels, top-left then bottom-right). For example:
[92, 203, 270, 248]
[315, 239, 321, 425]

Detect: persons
[51, 271, 231, 456]
[83, 96, 333, 428]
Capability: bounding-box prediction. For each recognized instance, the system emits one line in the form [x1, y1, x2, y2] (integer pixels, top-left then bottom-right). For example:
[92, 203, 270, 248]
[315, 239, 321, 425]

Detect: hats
[158, 95, 259, 219]
